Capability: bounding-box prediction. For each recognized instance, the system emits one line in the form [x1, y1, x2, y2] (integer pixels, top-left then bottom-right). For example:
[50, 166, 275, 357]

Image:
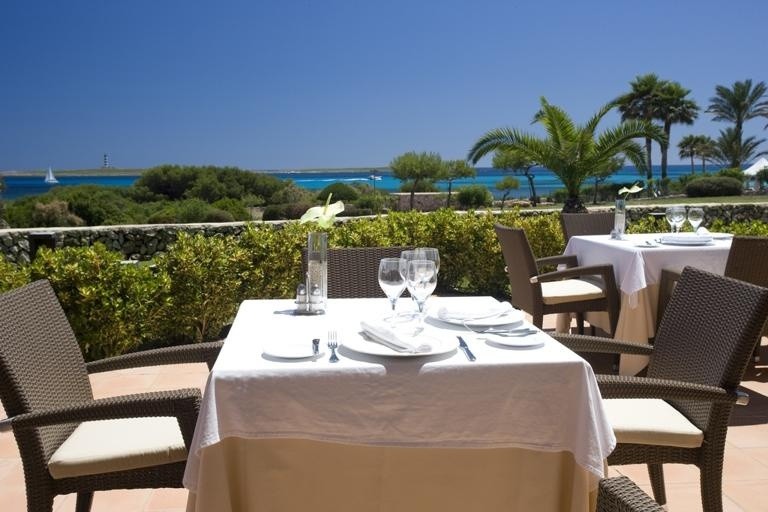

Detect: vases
[615, 199, 625, 240]
[307, 231, 327, 315]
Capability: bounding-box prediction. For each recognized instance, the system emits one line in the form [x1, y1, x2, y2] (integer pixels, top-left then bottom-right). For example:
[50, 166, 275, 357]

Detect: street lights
[370, 168, 378, 197]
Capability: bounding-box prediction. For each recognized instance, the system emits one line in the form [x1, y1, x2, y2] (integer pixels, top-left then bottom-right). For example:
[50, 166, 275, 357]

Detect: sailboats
[44, 163, 60, 184]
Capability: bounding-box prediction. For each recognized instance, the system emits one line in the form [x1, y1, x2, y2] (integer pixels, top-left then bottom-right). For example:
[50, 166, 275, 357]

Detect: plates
[263, 302, 546, 362]
[634, 232, 734, 247]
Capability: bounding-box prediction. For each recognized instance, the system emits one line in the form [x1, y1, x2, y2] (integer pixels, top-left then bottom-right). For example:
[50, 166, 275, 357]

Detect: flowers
[618, 181, 644, 211]
[299, 193, 345, 264]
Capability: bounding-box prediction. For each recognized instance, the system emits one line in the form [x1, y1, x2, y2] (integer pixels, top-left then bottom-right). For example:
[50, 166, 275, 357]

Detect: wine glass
[378, 247, 440, 314]
[665, 206, 704, 232]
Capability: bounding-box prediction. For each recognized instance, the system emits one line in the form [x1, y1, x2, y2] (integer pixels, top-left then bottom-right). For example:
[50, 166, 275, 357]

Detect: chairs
[542, 266, 768, 512]
[595, 474, 668, 512]
[493, 223, 621, 362]
[648, 235, 768, 364]
[300, 246, 416, 297]
[0, 278, 224, 512]
[558, 212, 616, 336]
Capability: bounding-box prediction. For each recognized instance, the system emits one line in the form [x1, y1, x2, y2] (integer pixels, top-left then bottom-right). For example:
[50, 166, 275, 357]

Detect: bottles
[296, 271, 323, 314]
[612, 228, 622, 240]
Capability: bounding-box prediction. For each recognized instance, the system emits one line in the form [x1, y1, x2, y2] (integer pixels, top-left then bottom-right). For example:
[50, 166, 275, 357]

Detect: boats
[369, 175, 383, 181]
